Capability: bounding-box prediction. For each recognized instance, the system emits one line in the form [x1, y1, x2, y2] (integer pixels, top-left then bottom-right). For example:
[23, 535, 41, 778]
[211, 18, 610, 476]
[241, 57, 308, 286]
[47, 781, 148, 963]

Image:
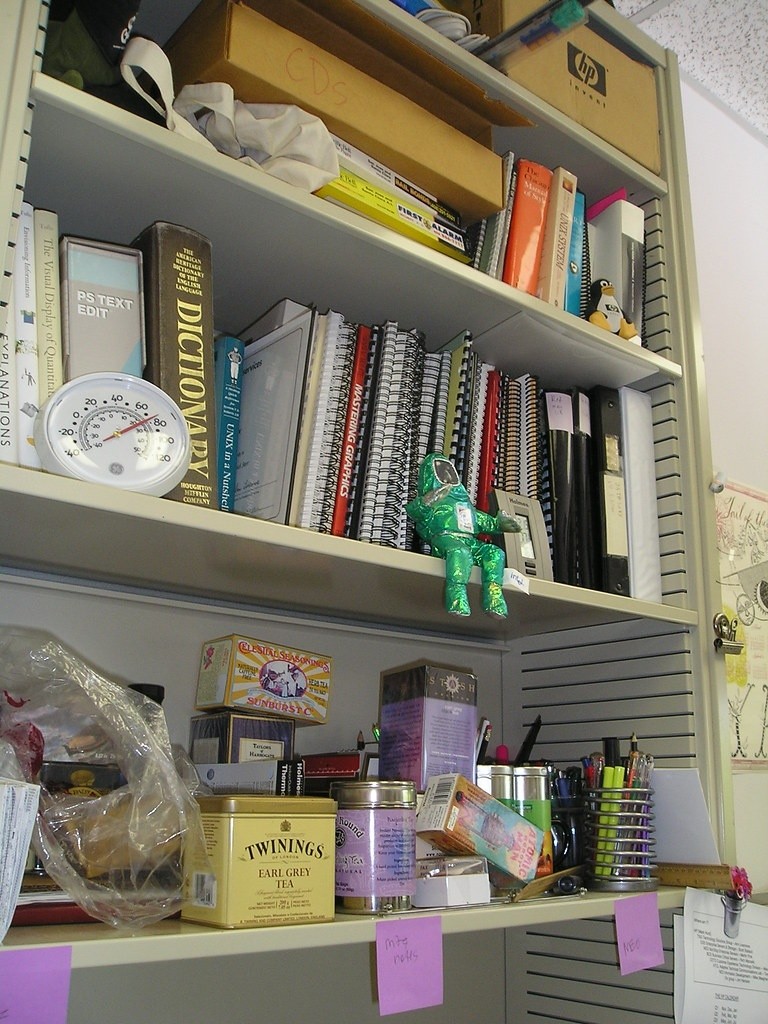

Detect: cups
[477, 767, 513, 800]
[513, 768, 549, 801]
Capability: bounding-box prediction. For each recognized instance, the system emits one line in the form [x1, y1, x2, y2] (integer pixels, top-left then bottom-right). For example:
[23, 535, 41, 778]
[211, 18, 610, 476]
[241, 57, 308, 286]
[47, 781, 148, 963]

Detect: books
[13, 202, 660, 608]
[107, 2, 645, 347]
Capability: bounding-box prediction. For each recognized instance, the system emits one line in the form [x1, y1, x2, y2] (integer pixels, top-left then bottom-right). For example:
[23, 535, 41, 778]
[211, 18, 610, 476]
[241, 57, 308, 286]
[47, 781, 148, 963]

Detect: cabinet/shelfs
[0, 0, 745, 1023]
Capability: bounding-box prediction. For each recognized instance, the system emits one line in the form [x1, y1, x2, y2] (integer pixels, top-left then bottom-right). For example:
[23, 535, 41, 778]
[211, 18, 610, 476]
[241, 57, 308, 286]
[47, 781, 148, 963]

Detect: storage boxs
[181, 634, 554, 932]
[438, 0, 660, 174]
[135, 0, 536, 222]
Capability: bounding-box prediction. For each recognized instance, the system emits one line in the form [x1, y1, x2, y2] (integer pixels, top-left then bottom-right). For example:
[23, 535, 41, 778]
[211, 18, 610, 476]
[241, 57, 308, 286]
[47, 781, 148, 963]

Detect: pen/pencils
[472, 710, 545, 766]
[353, 721, 383, 753]
[549, 728, 664, 896]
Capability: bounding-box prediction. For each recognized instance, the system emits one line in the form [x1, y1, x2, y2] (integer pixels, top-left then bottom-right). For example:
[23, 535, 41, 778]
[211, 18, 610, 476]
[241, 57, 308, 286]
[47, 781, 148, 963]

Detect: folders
[578, 376, 634, 602]
